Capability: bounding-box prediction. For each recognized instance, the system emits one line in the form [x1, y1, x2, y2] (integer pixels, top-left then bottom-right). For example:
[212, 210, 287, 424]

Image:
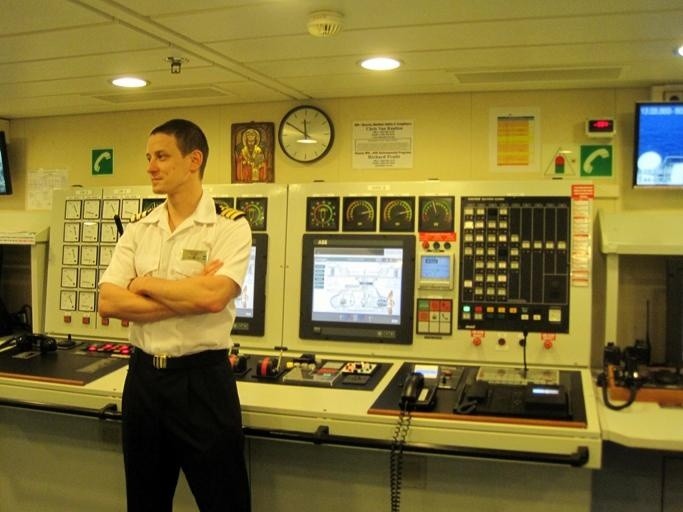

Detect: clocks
[277, 104, 336, 167]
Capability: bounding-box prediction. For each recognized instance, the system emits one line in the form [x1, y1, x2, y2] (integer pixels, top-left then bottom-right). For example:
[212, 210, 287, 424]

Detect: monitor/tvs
[299, 235, 413, 344]
[229, 233, 268, 336]
[0, 128, 12, 198]
[633, 101, 683, 189]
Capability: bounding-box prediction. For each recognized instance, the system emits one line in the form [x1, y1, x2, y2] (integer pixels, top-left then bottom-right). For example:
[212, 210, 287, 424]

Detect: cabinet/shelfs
[591, 208, 682, 453]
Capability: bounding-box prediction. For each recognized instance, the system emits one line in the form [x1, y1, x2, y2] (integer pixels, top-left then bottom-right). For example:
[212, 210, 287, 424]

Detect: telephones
[398, 373, 438, 411]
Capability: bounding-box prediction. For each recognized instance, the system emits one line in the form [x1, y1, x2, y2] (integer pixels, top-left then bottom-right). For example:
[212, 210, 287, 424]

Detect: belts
[134, 347, 229, 371]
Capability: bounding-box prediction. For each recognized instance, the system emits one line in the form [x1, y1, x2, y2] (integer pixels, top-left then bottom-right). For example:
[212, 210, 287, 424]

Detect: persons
[236, 130, 267, 181]
[98, 120, 252, 512]
